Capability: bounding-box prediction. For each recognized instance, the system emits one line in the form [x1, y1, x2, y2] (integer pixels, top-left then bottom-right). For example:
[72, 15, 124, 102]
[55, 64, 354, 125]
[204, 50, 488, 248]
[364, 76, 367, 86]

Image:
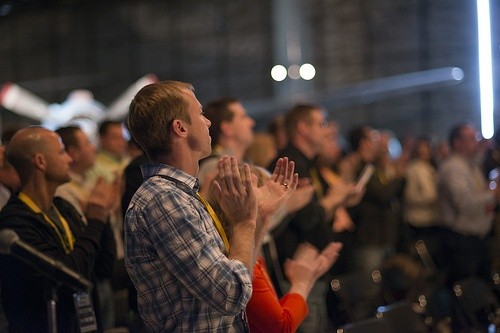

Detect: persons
[123, 80, 298, 333]
[54, 125, 124, 258]
[207, 163, 342, 333]
[197, 97, 255, 190]
[0, 127, 123, 333]
[267, 104, 361, 333]
[245, 112, 500, 253]
[93, 117, 134, 183]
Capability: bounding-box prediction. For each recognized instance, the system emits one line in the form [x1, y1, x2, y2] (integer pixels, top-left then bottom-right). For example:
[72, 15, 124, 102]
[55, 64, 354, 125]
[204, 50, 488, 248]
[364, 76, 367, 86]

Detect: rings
[280, 181, 290, 189]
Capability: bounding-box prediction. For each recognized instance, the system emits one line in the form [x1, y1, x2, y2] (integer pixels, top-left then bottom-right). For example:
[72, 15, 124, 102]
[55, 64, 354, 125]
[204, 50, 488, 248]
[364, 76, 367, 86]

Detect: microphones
[0, 229, 92, 294]
